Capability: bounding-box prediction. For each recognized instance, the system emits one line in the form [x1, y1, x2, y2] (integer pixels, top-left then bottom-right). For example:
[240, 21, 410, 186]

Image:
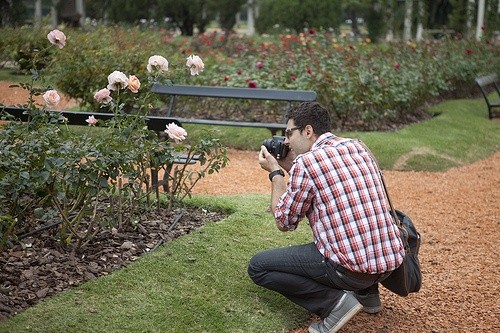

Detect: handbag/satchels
[379, 208, 424, 297]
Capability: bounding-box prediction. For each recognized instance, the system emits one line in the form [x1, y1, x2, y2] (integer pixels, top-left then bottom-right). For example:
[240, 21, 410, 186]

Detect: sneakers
[354, 293, 383, 313]
[307, 293, 363, 332]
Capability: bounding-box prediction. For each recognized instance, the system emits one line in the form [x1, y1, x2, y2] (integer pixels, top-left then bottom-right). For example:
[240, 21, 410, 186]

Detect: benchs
[150, 84, 317, 138]
[0, 107, 202, 192]
[474, 72, 500, 119]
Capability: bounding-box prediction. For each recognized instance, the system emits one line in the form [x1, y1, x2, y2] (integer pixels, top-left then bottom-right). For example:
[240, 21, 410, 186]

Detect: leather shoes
[329, 260, 391, 281]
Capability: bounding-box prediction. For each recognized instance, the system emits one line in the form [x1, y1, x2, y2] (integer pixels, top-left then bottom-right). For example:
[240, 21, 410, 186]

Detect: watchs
[269, 169, 285, 182]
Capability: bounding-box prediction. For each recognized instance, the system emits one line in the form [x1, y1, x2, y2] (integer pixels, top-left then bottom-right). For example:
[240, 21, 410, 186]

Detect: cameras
[262, 135, 290, 160]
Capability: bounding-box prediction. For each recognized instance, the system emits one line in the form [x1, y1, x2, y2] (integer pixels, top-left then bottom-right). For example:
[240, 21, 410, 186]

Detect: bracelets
[247, 102, 406, 333]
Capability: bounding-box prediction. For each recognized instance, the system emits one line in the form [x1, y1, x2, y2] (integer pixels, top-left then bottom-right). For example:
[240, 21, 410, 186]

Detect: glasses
[284, 126, 302, 138]
